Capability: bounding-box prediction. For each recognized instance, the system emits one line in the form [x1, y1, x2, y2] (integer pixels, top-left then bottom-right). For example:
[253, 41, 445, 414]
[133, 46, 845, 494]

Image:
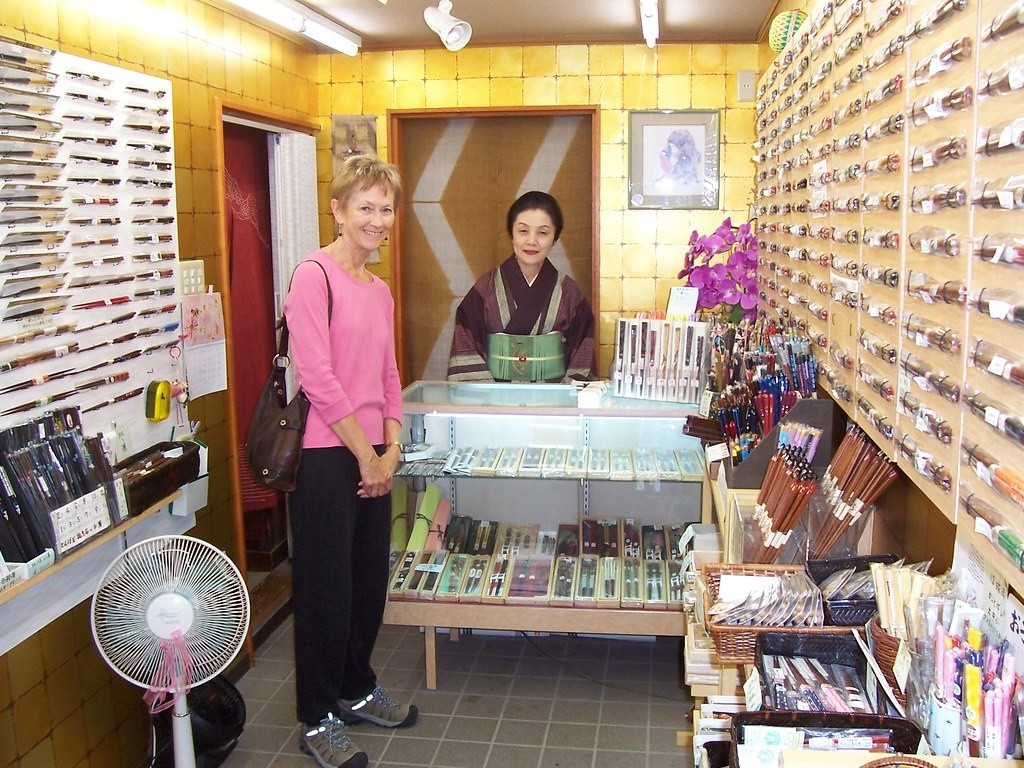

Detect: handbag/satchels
[246, 260, 333, 491]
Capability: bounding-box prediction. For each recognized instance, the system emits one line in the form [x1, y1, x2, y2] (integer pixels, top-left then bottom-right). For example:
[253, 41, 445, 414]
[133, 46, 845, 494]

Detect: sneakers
[299, 717, 369, 768]
[334, 682, 418, 728]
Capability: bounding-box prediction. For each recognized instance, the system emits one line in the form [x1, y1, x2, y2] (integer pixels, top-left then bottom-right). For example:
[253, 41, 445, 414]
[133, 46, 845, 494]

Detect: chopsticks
[712, 327, 898, 565]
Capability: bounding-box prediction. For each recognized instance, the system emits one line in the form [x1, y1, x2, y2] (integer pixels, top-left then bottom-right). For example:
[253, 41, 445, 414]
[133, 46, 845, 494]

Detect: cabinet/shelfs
[382, 378, 717, 691]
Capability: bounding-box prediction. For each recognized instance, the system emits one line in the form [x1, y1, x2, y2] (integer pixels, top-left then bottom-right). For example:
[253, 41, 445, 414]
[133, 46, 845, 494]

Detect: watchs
[385, 439, 403, 452]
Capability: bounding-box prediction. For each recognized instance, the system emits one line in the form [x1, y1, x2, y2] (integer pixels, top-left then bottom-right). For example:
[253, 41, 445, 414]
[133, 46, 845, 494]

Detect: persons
[446, 191, 595, 407]
[283, 155, 418, 768]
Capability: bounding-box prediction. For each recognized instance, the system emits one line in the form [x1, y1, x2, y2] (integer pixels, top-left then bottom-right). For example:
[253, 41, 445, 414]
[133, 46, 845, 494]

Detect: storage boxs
[108, 436, 200, 517]
[387, 515, 684, 611]
[471, 445, 706, 483]
[684, 491, 932, 768]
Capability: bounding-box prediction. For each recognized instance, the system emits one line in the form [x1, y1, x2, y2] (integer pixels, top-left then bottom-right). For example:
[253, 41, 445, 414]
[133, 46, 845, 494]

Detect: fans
[89, 534, 251, 768]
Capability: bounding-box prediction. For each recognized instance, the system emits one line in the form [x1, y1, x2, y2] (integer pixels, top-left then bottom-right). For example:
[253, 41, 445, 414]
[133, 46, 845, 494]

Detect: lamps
[223, 0, 362, 56]
[639, 0, 663, 48]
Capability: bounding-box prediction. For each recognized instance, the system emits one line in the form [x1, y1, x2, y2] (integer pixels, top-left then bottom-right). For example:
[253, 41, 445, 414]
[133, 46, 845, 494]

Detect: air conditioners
[423, 0, 472, 52]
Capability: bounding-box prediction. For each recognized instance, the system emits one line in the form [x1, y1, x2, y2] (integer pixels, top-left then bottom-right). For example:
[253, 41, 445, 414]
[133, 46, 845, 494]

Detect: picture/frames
[627, 109, 721, 210]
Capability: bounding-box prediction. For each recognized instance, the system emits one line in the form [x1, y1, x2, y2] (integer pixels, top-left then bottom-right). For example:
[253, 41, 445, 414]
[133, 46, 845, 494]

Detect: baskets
[702, 563, 869, 665]
[754, 632, 876, 714]
[804, 553, 901, 627]
[869, 615, 909, 704]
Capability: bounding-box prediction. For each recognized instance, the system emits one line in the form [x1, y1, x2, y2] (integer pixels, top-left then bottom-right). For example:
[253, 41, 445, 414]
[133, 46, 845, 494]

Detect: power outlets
[738, 70, 755, 102]
